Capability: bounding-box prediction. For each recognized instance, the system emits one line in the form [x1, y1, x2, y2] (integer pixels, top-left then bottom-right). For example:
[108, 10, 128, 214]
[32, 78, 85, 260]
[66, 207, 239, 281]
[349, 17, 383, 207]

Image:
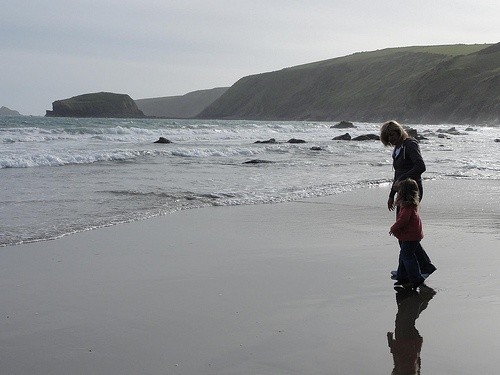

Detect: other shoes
[420, 264, 437, 280]
[390, 270, 398, 276]
[394, 277, 425, 287]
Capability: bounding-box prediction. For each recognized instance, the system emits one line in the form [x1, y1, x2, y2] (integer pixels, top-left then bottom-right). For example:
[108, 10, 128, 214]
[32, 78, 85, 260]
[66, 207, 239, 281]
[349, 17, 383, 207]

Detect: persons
[380, 120, 436, 290]
